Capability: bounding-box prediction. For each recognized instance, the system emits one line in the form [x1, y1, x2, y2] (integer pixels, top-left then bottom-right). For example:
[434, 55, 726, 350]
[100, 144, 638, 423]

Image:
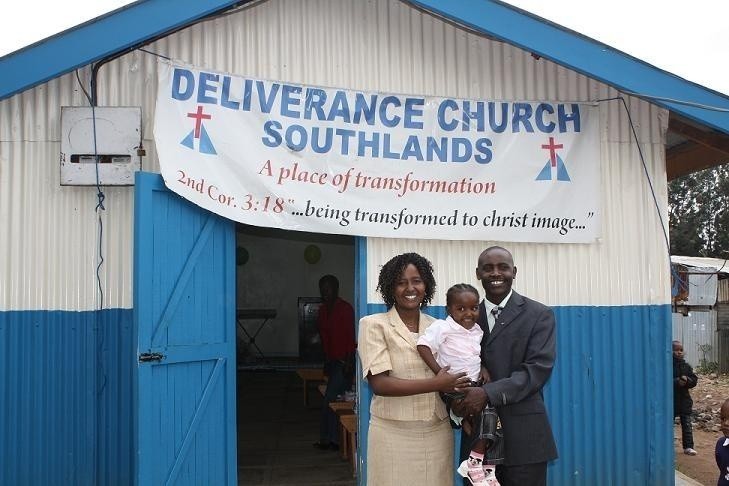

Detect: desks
[235, 309, 277, 367]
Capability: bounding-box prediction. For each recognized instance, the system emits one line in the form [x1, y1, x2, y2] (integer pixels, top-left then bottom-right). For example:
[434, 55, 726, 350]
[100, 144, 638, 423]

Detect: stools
[296, 367, 357, 479]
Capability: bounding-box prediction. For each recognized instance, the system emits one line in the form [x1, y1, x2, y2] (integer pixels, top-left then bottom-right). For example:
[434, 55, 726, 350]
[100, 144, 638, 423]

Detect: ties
[492, 306, 503, 323]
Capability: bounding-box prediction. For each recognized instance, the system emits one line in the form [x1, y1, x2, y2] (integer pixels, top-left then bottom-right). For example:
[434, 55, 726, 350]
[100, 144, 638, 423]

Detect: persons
[416, 283, 506, 486]
[672, 340, 698, 456]
[312, 274, 356, 451]
[714, 398, 729, 486]
[357, 252, 472, 486]
[449, 245, 558, 486]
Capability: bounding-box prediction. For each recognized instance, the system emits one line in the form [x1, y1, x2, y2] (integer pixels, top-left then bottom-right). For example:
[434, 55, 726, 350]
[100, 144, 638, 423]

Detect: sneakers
[684, 448, 697, 455]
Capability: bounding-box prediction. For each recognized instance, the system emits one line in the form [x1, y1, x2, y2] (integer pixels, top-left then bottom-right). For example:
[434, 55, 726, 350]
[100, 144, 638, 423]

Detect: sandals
[457, 460, 501, 486]
